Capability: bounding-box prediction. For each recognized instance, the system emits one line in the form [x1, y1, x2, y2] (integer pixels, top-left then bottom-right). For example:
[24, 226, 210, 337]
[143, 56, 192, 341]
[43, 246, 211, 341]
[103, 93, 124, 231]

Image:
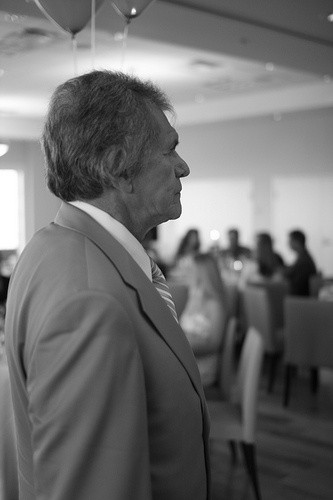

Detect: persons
[173, 230, 200, 260]
[4, 70, 211, 500]
[252, 233, 284, 277]
[283, 230, 318, 298]
[220, 229, 251, 260]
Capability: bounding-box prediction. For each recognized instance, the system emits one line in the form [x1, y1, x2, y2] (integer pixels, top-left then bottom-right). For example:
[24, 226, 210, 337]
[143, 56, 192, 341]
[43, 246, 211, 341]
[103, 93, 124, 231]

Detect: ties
[150, 257, 178, 326]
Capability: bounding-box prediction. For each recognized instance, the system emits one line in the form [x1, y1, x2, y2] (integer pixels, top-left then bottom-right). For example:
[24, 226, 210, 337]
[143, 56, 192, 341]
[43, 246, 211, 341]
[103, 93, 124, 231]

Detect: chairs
[206, 325, 266, 500]
[205, 317, 238, 402]
[243, 283, 284, 396]
[282, 295, 332, 406]
